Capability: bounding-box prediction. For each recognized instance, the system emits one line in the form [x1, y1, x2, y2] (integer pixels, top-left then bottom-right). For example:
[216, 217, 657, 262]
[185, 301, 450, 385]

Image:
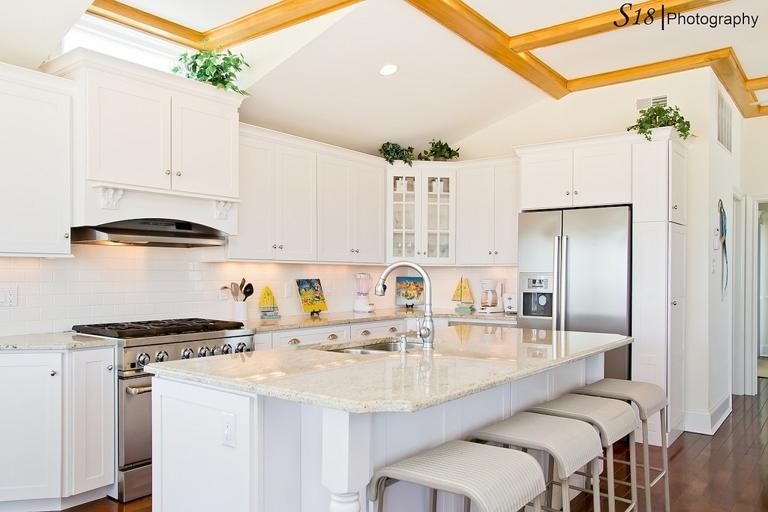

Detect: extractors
[72, 221, 229, 248]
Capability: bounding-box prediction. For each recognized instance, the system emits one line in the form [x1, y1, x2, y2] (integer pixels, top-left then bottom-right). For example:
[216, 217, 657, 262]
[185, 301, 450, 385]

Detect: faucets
[374, 260, 435, 351]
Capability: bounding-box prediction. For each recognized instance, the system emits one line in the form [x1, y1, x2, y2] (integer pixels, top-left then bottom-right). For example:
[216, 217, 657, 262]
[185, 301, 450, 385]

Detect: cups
[231, 301, 247, 325]
[396, 179, 408, 192]
[432, 180, 444, 193]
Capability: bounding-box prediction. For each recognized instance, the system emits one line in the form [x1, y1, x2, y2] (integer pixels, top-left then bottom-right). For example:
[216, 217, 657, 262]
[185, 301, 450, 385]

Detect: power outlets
[222, 411, 237, 447]
[7, 285, 17, 307]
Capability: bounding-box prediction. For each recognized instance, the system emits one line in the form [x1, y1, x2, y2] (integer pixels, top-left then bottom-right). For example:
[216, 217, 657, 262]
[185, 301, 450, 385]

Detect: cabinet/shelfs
[255, 323, 352, 349]
[404, 316, 448, 331]
[633, 137, 687, 225]
[2, 332, 116, 508]
[203, 126, 316, 262]
[392, 158, 455, 270]
[455, 156, 521, 268]
[521, 135, 635, 211]
[631, 219, 685, 447]
[0, 60, 73, 258]
[41, 51, 243, 215]
[316, 139, 392, 263]
[350, 317, 405, 344]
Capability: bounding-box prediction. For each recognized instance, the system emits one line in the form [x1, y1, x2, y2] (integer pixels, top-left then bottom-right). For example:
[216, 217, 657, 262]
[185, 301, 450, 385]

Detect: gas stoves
[73, 317, 254, 371]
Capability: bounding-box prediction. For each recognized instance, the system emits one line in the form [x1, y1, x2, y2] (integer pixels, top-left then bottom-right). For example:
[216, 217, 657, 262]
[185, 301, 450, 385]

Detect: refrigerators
[516, 328, 589, 368]
[516, 206, 632, 379]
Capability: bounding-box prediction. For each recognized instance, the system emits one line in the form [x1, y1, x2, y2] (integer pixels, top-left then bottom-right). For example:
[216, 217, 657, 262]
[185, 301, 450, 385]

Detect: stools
[571, 378, 674, 511]
[531, 393, 640, 511]
[466, 411, 603, 511]
[367, 440, 547, 512]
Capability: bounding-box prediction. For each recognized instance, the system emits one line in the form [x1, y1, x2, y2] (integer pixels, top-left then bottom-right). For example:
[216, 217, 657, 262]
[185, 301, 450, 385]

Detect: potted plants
[418, 140, 458, 162]
[628, 103, 690, 144]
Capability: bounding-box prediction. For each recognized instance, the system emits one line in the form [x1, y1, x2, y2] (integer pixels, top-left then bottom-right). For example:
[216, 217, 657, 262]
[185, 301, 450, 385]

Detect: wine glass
[397, 241, 413, 257]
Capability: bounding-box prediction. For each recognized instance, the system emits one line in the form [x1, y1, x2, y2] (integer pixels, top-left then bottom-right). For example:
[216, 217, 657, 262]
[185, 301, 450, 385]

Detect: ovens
[115, 376, 151, 503]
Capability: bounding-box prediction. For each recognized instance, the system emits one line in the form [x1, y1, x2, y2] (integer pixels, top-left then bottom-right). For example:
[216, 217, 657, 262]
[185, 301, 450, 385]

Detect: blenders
[352, 273, 375, 314]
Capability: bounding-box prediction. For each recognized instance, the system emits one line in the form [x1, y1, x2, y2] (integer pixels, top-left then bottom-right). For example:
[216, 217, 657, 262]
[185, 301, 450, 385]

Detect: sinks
[356, 339, 424, 352]
[324, 347, 385, 356]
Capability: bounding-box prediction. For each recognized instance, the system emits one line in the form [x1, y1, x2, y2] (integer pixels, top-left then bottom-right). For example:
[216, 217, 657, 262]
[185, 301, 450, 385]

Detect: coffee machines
[479, 278, 504, 313]
